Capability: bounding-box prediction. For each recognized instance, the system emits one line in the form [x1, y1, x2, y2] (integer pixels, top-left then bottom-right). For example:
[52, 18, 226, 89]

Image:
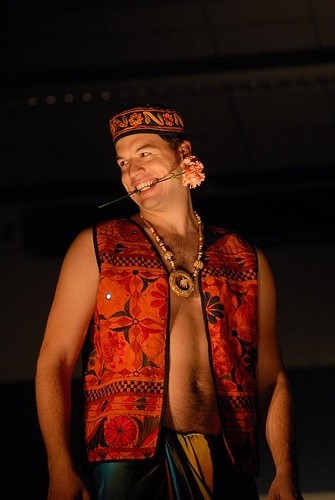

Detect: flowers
[99, 155, 206, 209]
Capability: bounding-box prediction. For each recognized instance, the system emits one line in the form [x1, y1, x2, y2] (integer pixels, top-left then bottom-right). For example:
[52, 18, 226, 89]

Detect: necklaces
[137, 211, 205, 297]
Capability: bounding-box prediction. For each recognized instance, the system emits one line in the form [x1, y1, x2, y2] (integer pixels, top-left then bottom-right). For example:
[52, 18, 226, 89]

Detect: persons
[35, 104, 303, 500]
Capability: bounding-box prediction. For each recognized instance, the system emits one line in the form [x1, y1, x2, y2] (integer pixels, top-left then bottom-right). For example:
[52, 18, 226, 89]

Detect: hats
[108, 105, 200, 145]
